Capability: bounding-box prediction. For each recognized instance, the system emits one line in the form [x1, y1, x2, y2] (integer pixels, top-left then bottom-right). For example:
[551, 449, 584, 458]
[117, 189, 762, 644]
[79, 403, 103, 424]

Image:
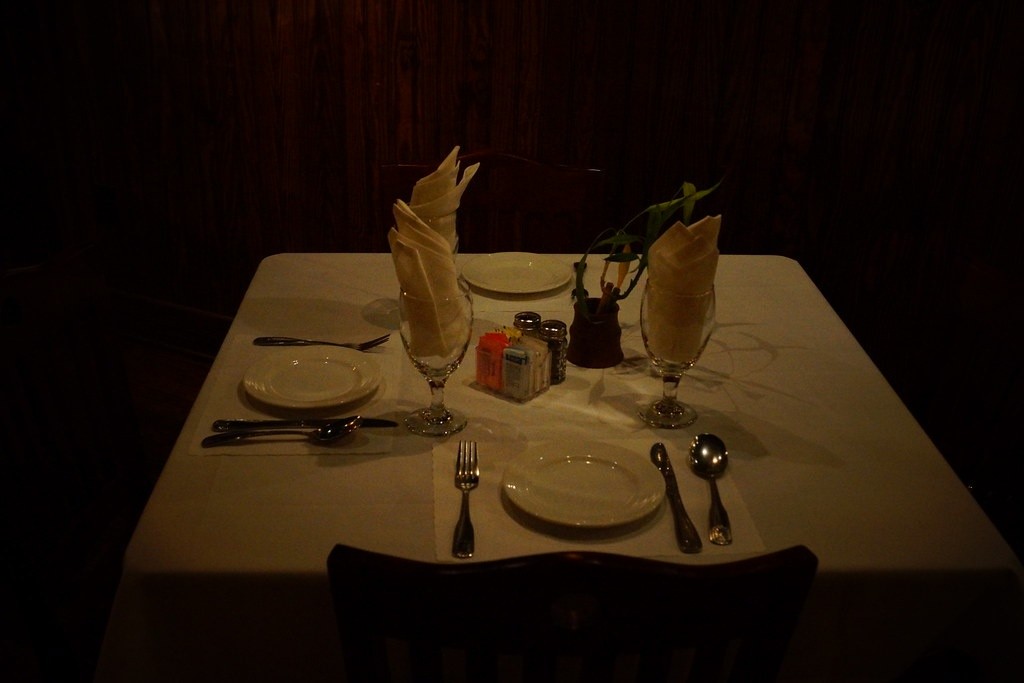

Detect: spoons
[688, 433, 733, 545]
[201, 415, 366, 447]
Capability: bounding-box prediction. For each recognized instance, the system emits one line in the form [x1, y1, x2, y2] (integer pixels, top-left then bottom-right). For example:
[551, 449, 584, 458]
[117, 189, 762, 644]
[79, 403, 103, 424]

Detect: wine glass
[398, 281, 473, 436]
[635, 278, 718, 430]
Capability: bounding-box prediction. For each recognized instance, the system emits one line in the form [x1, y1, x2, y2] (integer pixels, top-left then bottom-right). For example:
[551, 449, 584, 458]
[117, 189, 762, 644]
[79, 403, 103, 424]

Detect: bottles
[537, 320, 567, 386]
[513, 312, 541, 341]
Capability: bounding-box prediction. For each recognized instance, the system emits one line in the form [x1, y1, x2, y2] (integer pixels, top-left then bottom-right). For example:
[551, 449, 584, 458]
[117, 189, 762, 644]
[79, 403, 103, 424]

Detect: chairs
[379, 147, 606, 251]
[326, 544, 821, 683]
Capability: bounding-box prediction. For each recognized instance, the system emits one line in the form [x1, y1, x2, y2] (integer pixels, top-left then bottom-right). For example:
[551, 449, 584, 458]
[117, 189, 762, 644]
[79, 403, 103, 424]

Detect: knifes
[212, 418, 398, 432]
[650, 443, 703, 554]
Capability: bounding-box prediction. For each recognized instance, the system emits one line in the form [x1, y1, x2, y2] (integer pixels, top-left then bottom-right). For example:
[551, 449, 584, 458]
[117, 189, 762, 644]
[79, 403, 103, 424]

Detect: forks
[253, 334, 391, 351]
[452, 440, 480, 559]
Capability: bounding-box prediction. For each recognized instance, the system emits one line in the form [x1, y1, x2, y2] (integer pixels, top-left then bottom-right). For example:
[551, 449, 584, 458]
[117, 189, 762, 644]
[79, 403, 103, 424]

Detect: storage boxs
[473, 347, 553, 401]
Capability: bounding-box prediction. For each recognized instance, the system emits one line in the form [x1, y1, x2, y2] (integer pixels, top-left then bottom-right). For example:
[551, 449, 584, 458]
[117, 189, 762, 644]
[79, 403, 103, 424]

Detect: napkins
[383, 145, 481, 357]
[644, 214, 723, 366]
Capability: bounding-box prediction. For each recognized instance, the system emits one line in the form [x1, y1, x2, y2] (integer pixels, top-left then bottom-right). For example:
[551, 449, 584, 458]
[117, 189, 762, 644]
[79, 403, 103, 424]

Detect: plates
[504, 441, 666, 530]
[242, 345, 382, 410]
[461, 252, 573, 293]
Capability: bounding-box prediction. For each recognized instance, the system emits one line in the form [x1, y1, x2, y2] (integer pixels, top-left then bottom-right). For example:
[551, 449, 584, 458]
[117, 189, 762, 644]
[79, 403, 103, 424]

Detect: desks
[100, 254, 1024, 683]
